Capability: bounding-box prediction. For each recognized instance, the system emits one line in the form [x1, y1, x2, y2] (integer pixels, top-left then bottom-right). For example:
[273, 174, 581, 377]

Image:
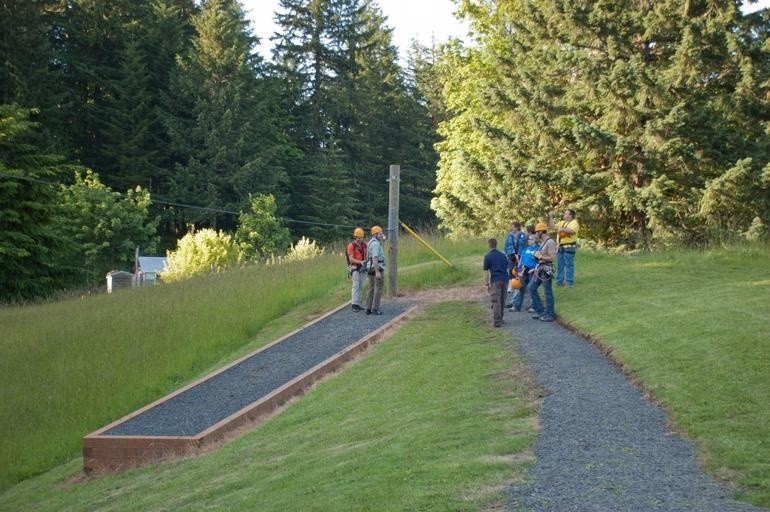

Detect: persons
[347, 226, 367, 312]
[482, 238, 507, 327]
[548, 209, 580, 288]
[502, 221, 558, 322]
[365, 225, 386, 314]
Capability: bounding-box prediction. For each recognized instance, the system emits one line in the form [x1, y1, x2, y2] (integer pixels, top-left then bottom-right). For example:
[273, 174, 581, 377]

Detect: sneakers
[494, 318, 505, 328]
[526, 304, 555, 322]
[351, 304, 383, 315]
[504, 302, 521, 312]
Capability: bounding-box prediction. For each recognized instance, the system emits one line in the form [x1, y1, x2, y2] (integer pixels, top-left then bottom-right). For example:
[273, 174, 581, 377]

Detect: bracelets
[485, 283, 490, 287]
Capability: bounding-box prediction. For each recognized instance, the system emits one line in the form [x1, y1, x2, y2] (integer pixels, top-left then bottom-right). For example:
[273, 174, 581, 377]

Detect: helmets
[511, 278, 522, 289]
[353, 227, 366, 238]
[535, 223, 548, 232]
[511, 267, 518, 277]
[370, 226, 383, 235]
